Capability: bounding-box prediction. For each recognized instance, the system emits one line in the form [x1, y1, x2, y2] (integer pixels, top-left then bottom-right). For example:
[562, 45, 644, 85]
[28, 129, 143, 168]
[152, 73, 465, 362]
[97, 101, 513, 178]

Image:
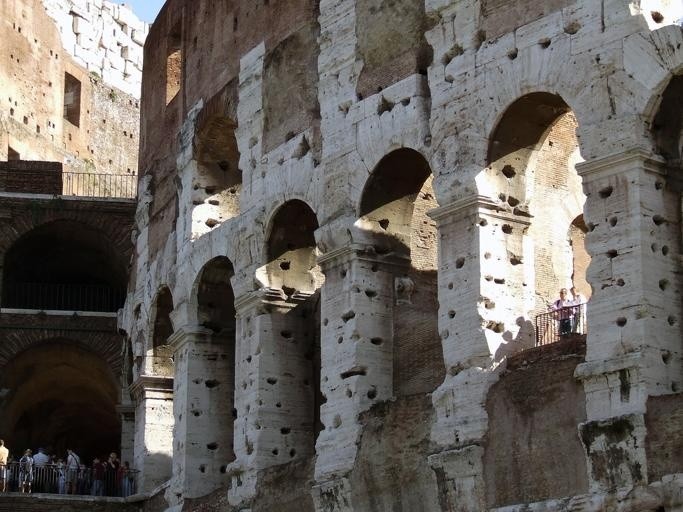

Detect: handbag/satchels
[77, 465, 81, 473]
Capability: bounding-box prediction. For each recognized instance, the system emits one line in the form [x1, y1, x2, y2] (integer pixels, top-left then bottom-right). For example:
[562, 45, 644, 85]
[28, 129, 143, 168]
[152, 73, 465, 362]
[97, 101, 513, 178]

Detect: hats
[39, 448, 44, 452]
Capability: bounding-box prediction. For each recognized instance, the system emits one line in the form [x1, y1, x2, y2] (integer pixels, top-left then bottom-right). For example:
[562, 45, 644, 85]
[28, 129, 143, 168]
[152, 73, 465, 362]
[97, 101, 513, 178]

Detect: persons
[0, 438, 131, 497]
[567, 286, 587, 335]
[547, 288, 578, 339]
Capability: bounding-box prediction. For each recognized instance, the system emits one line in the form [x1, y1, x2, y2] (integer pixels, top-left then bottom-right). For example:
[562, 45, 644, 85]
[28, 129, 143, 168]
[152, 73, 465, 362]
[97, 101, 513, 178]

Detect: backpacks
[20, 458, 30, 473]
